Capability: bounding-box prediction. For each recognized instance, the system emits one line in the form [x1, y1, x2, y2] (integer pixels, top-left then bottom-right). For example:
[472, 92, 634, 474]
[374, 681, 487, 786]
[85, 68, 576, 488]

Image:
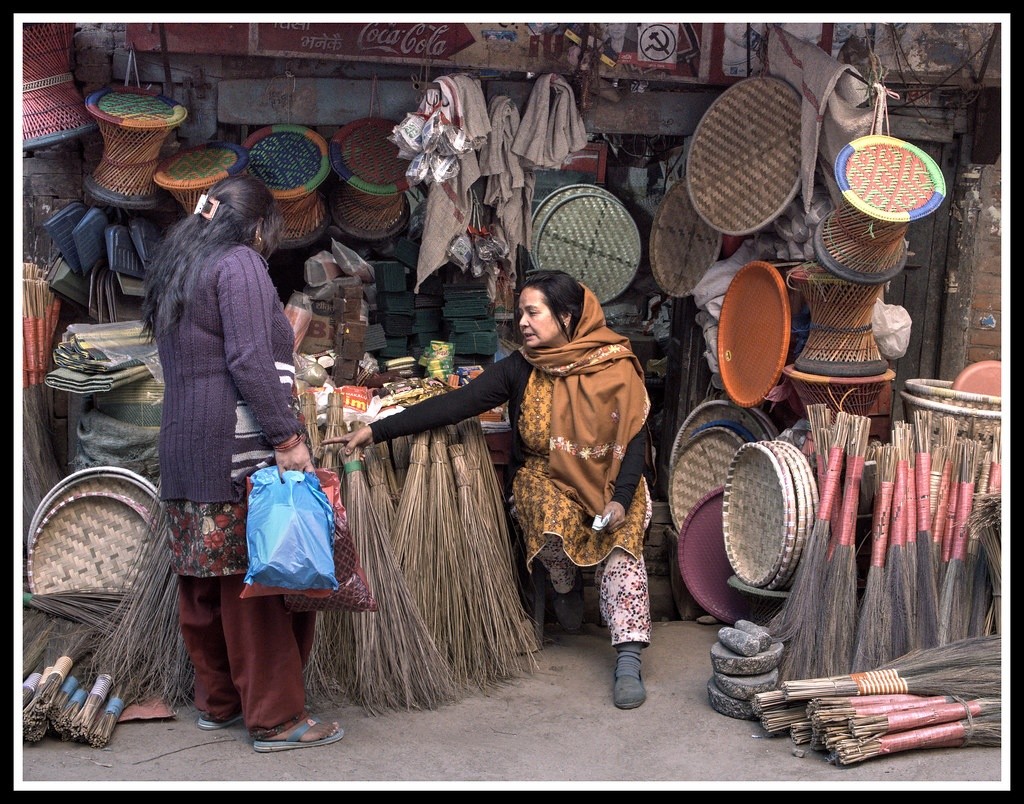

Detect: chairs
[524, 557, 607, 644]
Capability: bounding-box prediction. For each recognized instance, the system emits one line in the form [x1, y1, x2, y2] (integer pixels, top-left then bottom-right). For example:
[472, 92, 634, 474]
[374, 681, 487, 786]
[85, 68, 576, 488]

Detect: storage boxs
[300, 286, 508, 423]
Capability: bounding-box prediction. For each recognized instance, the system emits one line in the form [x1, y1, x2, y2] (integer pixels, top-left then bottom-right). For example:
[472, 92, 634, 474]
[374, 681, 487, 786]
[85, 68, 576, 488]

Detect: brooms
[749, 397, 1001, 766]
[20, 258, 536, 753]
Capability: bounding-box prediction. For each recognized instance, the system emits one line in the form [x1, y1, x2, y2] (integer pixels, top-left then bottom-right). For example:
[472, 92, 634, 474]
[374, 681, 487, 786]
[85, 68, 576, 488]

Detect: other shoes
[554, 578, 584, 633]
[614, 666, 646, 709]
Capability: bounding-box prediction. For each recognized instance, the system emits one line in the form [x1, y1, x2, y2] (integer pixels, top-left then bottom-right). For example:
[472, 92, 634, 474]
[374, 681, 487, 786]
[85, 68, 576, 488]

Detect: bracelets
[274, 433, 304, 452]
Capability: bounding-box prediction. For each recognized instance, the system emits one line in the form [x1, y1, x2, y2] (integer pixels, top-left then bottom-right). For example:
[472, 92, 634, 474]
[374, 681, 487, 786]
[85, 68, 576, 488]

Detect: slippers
[254, 716, 344, 752]
[197, 714, 243, 730]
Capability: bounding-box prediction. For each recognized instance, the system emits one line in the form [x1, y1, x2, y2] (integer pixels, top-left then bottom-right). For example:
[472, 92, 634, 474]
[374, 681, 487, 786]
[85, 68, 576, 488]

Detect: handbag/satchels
[241, 466, 379, 613]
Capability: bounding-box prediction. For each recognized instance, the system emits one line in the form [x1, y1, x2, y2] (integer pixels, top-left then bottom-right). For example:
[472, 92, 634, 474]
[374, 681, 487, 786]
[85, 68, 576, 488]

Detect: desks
[485, 430, 513, 496]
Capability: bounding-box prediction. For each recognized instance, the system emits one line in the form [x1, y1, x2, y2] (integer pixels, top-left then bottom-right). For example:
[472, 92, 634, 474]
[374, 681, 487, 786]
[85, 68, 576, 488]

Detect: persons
[140, 173, 344, 752]
[320, 270, 653, 708]
